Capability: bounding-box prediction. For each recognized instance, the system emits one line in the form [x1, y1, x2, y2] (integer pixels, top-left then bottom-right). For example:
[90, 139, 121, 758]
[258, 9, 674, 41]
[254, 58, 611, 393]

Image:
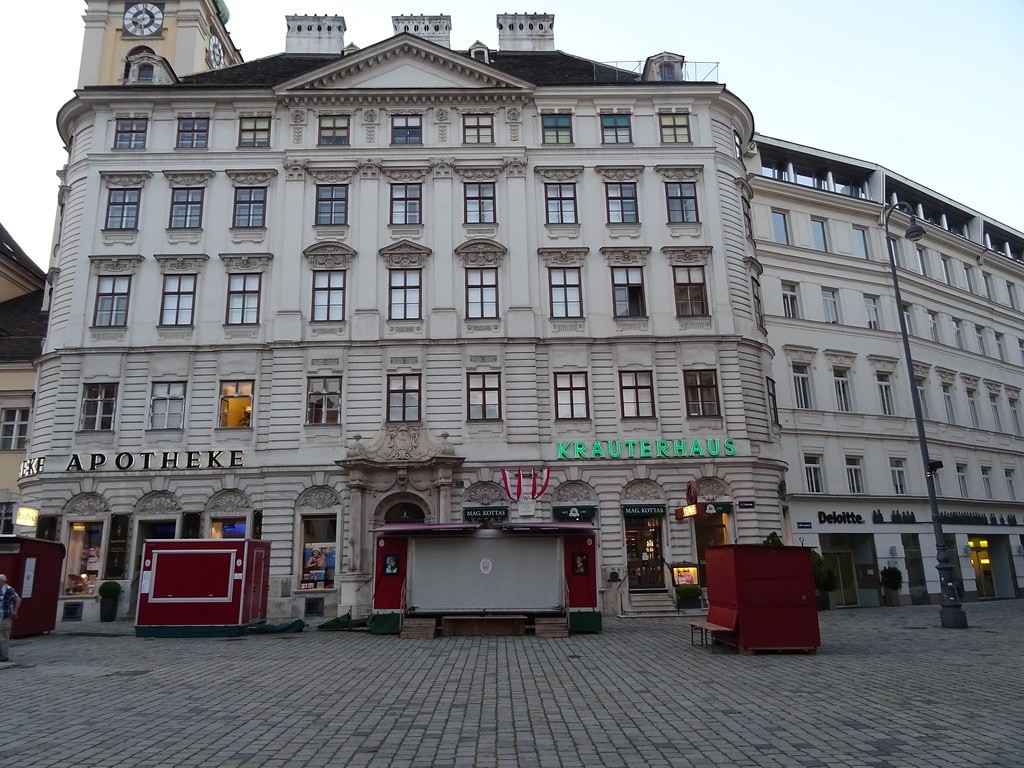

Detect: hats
[312, 547, 320, 553]
[0, 574, 6, 580]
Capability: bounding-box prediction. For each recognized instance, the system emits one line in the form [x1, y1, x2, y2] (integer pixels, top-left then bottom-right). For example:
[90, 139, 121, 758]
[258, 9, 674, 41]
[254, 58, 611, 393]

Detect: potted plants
[98, 582, 121, 621]
[675, 584, 702, 609]
[880, 565, 903, 607]
[812, 549, 838, 611]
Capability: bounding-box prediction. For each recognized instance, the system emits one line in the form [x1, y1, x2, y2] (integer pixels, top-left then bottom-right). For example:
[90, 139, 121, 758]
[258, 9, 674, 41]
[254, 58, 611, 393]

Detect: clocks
[208, 35, 224, 68]
[123, 2, 164, 38]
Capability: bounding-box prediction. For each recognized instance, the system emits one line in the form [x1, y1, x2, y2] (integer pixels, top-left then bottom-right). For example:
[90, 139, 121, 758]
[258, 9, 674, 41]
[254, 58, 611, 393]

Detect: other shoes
[0, 658, 9, 662]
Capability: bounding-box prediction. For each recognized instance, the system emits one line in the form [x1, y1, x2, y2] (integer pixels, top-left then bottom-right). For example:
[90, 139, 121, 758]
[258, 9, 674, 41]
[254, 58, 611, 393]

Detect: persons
[386, 557, 397, 573]
[574, 556, 584, 572]
[0, 573, 23, 662]
[307, 547, 331, 570]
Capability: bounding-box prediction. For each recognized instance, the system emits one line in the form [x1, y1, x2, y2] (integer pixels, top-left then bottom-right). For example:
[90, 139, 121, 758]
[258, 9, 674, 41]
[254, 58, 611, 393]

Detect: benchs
[686, 605, 738, 654]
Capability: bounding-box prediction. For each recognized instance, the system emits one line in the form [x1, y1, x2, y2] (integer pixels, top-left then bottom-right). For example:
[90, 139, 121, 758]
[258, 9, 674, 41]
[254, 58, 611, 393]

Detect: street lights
[885, 201, 969, 629]
